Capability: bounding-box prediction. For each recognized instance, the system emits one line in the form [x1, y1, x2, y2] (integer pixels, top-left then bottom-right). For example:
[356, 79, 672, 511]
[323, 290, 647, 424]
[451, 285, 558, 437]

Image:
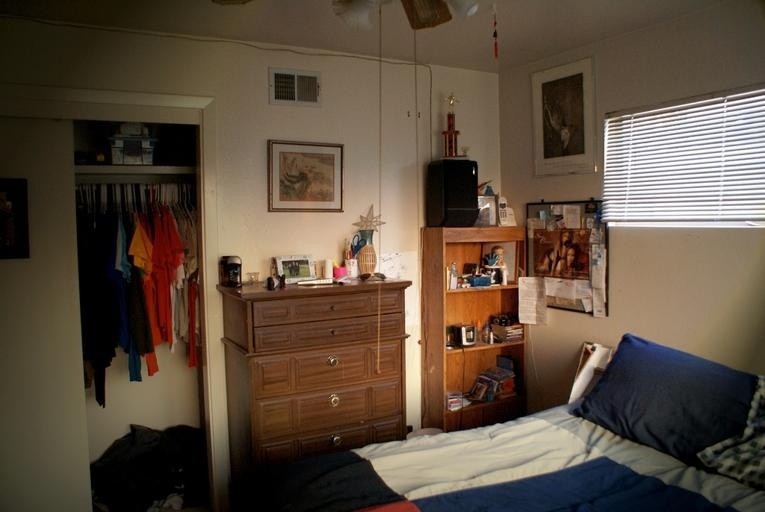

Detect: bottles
[450, 262, 458, 290]
[476, 319, 494, 345]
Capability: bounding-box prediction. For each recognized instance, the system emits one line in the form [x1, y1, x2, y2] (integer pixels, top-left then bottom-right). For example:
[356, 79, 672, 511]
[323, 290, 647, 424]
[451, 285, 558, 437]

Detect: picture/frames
[264, 137, 344, 216]
[522, 199, 610, 319]
[275, 254, 317, 286]
[527, 54, 598, 178]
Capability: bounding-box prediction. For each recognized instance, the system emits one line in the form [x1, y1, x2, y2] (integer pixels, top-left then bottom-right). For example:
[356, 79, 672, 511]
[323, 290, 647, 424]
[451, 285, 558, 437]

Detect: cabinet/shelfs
[424, 226, 529, 436]
[218, 273, 413, 483]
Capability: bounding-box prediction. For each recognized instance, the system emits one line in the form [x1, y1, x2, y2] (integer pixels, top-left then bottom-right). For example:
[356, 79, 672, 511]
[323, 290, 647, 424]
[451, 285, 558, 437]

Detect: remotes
[297, 279, 333, 285]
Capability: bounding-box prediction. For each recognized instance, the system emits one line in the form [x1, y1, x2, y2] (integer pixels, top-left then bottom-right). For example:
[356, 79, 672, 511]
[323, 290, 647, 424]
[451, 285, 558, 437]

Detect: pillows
[570, 329, 765, 489]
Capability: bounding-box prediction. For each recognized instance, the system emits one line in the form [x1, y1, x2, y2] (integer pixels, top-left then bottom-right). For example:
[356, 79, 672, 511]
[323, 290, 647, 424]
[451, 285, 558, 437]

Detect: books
[492, 322, 524, 342]
[448, 355, 520, 409]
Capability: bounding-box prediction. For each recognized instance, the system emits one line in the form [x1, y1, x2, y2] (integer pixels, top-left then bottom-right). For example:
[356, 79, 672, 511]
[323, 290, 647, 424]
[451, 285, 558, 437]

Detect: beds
[228, 338, 765, 511]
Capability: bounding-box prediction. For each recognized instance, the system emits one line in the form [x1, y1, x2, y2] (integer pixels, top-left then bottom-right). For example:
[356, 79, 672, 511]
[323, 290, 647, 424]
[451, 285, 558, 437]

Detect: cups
[345, 259, 358, 277]
[480, 265, 500, 286]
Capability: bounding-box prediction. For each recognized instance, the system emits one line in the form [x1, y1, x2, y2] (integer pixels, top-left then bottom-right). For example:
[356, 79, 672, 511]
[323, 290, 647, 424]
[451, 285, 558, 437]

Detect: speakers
[427, 160, 478, 227]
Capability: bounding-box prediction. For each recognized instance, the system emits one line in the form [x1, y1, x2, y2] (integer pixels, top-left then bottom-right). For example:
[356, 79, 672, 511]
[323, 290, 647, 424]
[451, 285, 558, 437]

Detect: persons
[555, 244, 581, 272]
[535, 229, 573, 271]
